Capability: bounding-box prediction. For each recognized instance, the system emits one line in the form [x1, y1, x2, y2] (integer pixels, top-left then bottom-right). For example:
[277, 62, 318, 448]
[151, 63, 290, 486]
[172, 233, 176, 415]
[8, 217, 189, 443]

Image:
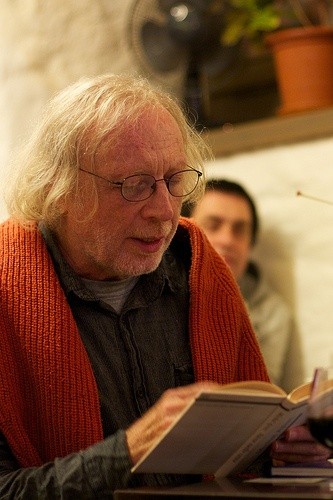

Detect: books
[131, 379, 333, 478]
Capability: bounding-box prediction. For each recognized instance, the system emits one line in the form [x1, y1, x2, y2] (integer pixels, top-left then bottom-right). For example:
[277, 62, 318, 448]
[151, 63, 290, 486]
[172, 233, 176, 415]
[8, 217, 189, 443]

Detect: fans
[126, 0, 247, 129]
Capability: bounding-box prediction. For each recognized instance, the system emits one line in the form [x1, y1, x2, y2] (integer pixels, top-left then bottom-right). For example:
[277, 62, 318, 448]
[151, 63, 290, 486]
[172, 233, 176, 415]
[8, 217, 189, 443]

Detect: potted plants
[229, 4, 333, 114]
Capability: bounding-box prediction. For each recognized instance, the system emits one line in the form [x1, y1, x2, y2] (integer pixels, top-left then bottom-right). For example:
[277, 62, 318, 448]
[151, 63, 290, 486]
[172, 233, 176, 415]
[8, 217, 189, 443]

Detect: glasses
[79, 164, 202, 203]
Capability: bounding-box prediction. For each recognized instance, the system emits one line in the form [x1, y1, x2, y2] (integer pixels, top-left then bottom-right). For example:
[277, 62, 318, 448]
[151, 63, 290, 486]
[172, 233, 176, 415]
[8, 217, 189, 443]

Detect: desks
[112, 468, 333, 500]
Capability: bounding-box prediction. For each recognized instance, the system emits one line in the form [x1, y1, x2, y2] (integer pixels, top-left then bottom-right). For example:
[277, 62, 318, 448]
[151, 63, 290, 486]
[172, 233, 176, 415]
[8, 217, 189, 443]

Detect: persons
[0, 75, 333, 500]
[181, 179, 307, 401]
